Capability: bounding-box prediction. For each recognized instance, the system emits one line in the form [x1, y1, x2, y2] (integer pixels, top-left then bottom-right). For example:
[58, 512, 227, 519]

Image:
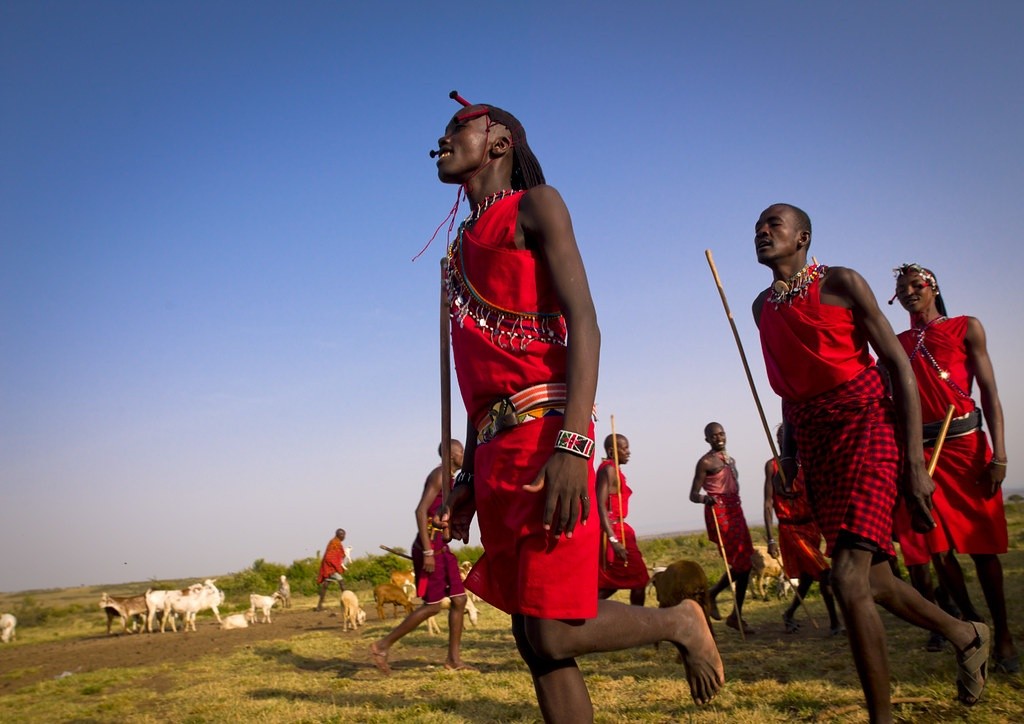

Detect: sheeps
[425, 593, 481, 636]
[340, 590, 366, 631]
[374, 584, 415, 621]
[652, 560, 723, 657]
[219, 592, 282, 630]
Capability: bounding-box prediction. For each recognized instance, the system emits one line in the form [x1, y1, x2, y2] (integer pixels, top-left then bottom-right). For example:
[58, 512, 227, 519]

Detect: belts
[470, 386, 600, 447]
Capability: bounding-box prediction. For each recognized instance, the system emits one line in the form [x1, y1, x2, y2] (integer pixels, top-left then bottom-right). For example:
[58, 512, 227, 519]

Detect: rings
[581, 496, 591, 501]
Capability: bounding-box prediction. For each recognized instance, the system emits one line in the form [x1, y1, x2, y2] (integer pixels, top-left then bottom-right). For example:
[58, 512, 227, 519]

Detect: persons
[367, 438, 481, 678]
[596, 433, 650, 604]
[316, 529, 348, 611]
[430, 90, 726, 724]
[763, 422, 960, 651]
[877, 262, 1020, 674]
[689, 421, 754, 634]
[752, 203, 991, 724]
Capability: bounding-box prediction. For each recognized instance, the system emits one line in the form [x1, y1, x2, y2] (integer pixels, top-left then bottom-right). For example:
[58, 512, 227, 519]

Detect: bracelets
[989, 455, 1008, 466]
[553, 430, 594, 458]
[768, 538, 777, 544]
[422, 549, 434, 557]
[609, 535, 618, 543]
[778, 456, 795, 462]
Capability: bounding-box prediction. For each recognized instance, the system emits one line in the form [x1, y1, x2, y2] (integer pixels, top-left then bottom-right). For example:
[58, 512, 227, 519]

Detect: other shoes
[725, 616, 752, 634]
[708, 596, 721, 619]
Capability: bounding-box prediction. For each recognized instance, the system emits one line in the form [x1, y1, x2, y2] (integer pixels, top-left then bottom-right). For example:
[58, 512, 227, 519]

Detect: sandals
[783, 612, 797, 634]
[992, 651, 1023, 676]
[958, 622, 989, 708]
[926, 633, 945, 651]
[830, 623, 847, 637]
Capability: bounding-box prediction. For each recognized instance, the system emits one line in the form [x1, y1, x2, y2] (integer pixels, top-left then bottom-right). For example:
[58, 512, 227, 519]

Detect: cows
[99, 579, 224, 634]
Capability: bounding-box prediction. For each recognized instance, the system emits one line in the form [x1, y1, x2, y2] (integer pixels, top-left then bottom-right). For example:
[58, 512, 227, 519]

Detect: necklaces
[771, 263, 810, 300]
